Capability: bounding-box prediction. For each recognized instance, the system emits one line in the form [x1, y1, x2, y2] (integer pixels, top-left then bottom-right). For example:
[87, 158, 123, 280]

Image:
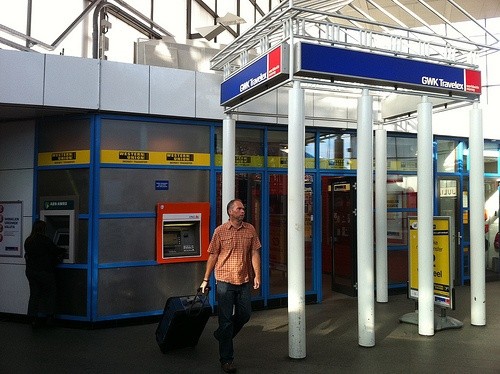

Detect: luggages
[155, 285, 214, 352]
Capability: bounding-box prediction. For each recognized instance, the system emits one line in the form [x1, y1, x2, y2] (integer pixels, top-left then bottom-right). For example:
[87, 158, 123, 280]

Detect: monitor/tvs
[164, 231, 181, 246]
[55, 233, 69, 248]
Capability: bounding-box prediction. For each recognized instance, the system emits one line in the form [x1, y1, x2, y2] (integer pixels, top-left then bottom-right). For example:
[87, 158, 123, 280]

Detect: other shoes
[214, 328, 220, 341]
[221, 361, 237, 373]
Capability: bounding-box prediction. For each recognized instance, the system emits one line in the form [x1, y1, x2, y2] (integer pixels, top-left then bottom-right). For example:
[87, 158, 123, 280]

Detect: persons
[200, 199, 263, 374]
[24, 220, 69, 328]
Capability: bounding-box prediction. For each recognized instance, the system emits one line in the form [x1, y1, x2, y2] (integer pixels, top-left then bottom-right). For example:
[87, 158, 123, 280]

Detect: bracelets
[203, 279, 210, 283]
